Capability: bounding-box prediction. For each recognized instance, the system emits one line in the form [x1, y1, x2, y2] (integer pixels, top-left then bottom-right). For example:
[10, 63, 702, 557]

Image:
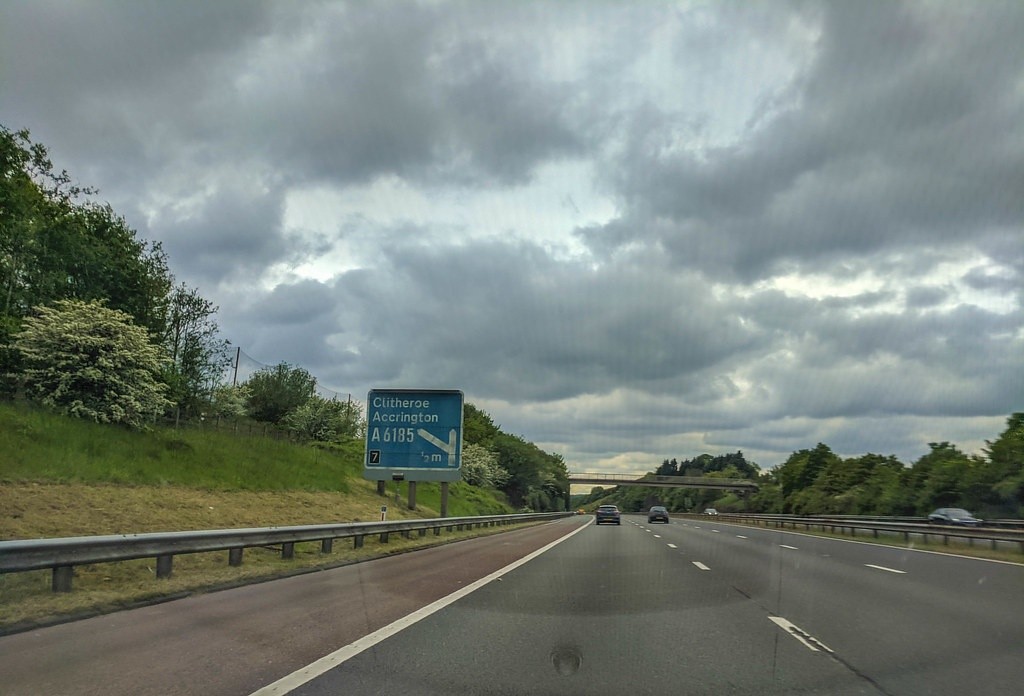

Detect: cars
[928, 508, 984, 527]
[648, 506, 669, 524]
[703, 509, 718, 516]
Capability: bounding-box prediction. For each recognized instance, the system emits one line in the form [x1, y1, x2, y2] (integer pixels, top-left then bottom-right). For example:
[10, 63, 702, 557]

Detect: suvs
[596, 505, 620, 525]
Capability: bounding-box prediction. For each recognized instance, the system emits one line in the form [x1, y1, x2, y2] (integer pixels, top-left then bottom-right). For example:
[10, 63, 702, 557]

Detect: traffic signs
[364, 388, 465, 482]
